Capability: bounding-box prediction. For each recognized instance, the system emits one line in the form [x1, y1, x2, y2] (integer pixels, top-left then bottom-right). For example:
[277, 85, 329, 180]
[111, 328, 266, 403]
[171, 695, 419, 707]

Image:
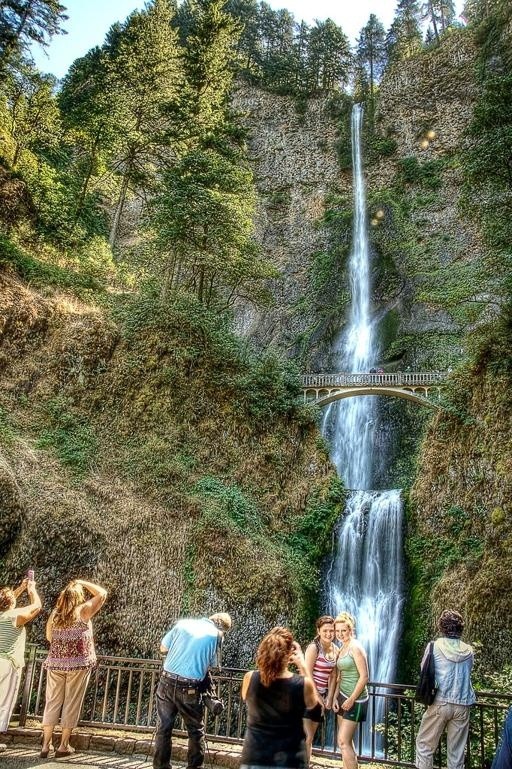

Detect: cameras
[201, 690, 224, 716]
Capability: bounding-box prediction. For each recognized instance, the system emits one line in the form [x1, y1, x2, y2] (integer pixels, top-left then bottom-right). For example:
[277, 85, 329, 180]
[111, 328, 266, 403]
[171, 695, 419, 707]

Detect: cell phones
[27, 569, 34, 582]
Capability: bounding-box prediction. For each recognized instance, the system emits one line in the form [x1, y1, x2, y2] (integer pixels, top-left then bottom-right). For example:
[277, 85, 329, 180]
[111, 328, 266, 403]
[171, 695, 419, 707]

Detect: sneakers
[0, 744, 7, 753]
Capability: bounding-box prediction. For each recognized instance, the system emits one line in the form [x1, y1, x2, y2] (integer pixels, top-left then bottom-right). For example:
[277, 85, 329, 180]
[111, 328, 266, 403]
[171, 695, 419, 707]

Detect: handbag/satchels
[414, 641, 439, 705]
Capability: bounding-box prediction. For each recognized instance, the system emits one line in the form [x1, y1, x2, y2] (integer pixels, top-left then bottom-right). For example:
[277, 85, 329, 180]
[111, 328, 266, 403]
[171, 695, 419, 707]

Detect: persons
[1, 577, 40, 751]
[39, 575, 110, 760]
[330, 611, 369, 768]
[236, 625, 319, 768]
[300, 614, 340, 767]
[150, 608, 234, 768]
[412, 609, 475, 768]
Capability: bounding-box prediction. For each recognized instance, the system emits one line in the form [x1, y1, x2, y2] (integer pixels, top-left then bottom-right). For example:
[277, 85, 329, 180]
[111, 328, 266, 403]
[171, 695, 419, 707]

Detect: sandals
[39, 744, 54, 758]
[55, 744, 75, 758]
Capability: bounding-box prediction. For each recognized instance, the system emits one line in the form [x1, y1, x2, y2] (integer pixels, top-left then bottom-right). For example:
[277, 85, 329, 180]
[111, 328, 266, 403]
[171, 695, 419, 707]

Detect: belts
[160, 669, 203, 684]
